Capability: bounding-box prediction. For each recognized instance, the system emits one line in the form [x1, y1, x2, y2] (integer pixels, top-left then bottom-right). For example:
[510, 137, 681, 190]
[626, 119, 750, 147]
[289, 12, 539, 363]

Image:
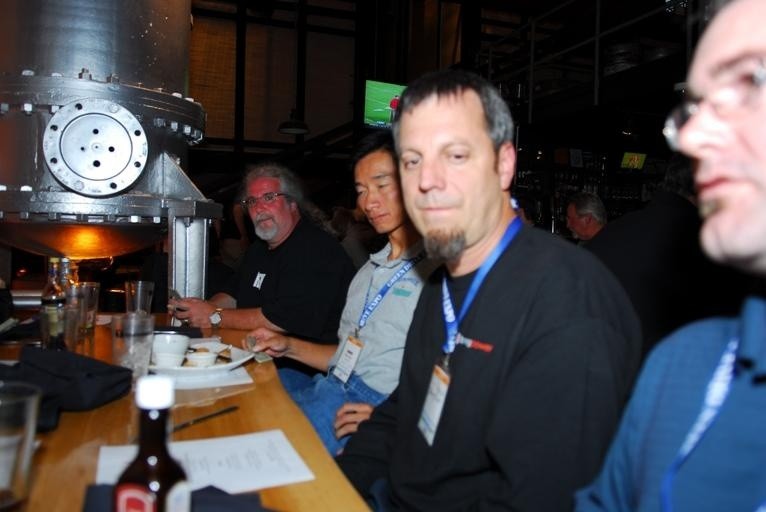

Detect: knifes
[172, 405, 237, 431]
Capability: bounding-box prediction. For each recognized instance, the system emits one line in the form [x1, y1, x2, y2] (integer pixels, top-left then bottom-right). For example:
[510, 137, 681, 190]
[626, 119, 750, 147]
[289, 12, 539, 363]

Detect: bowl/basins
[184, 351, 218, 367]
[154, 353, 185, 367]
[151, 334, 188, 355]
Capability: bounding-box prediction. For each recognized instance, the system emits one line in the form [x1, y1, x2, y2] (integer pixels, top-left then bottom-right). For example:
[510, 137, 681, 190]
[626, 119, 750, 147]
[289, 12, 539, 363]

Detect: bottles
[112, 375, 192, 512]
[0, 334, 22, 368]
[39, 256, 72, 350]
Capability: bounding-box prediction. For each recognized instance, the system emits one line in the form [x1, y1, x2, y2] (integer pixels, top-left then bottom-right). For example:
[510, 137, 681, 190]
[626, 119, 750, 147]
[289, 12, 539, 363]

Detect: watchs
[209, 308, 222, 331]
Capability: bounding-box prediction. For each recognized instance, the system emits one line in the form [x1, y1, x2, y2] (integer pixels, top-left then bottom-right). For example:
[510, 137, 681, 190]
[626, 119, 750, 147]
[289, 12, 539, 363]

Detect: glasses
[246, 193, 287, 207]
[662, 70, 766, 150]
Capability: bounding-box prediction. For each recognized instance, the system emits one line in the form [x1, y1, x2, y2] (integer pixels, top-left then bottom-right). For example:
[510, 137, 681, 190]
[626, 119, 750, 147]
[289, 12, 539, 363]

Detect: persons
[168, 172, 351, 395]
[240, 139, 429, 460]
[573, 2, 766, 511]
[332, 69, 652, 512]
[567, 187, 621, 273]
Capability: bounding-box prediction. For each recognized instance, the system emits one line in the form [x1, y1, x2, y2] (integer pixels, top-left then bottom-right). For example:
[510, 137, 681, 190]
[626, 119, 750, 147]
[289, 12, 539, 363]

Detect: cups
[124, 281, 153, 318]
[67, 281, 99, 332]
[0, 384, 43, 512]
[111, 314, 155, 381]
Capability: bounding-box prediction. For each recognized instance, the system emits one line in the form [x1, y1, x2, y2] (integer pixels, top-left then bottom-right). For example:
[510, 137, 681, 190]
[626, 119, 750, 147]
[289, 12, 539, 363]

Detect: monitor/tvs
[363, 79, 409, 130]
[620, 151, 649, 170]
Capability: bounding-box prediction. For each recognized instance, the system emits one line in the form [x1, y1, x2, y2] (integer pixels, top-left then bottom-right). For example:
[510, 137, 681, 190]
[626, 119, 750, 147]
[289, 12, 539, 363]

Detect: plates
[149, 344, 255, 374]
